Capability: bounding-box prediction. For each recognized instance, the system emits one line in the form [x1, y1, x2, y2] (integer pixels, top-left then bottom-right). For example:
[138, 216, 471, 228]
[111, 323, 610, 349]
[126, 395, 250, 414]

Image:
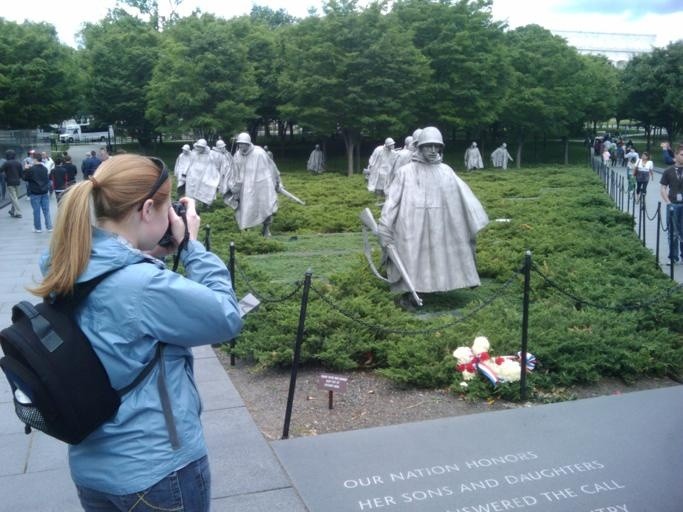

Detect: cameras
[158, 200, 186, 247]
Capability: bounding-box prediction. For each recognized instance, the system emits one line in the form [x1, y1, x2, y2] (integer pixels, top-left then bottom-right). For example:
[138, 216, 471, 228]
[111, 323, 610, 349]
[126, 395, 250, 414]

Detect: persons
[0, 151, 239, 510]
[307, 143, 325, 175]
[369, 131, 515, 208]
[659, 141, 683, 265]
[0, 149, 22, 218]
[19, 153, 52, 232]
[22, 146, 105, 209]
[173, 132, 285, 236]
[378, 126, 489, 295]
[592, 122, 655, 204]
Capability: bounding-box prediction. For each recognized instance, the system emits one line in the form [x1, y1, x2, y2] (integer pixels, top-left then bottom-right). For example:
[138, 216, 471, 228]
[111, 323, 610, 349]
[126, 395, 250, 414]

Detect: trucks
[58, 122, 116, 145]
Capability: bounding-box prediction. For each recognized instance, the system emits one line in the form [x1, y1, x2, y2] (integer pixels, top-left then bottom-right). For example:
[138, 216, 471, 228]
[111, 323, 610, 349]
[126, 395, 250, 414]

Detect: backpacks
[0, 260, 182, 452]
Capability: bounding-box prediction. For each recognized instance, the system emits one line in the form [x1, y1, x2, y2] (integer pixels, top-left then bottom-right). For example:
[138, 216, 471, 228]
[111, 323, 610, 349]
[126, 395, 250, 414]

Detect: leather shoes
[14, 215, 22, 218]
[8, 210, 13, 217]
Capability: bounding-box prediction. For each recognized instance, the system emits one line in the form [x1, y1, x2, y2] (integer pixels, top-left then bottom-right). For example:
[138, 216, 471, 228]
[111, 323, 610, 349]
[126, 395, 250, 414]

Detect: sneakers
[32, 228, 42, 233]
[48, 229, 53, 232]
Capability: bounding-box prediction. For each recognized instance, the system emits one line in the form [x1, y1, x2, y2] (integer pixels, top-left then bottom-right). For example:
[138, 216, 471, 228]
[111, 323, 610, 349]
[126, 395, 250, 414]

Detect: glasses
[138, 155, 169, 212]
